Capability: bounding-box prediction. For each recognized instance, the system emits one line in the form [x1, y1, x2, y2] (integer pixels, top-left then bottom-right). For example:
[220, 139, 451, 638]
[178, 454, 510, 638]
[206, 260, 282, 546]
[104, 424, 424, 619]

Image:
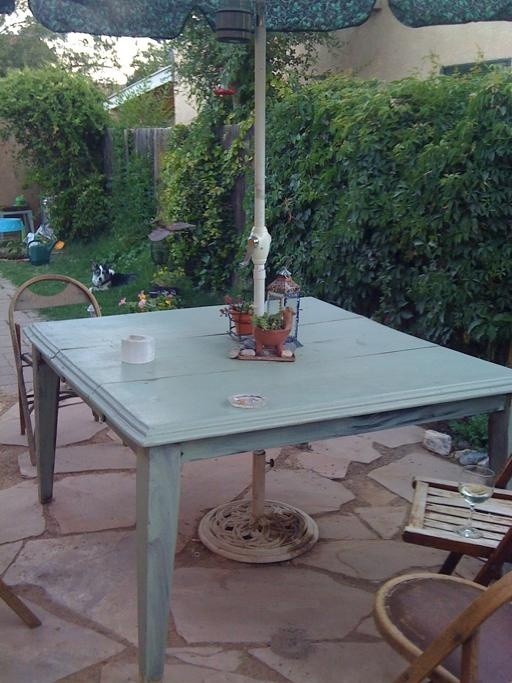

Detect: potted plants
[223, 255, 294, 358]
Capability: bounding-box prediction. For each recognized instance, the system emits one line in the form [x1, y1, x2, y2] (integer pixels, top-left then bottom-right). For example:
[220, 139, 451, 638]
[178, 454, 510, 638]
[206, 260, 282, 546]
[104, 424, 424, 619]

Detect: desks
[22, 296, 511, 682]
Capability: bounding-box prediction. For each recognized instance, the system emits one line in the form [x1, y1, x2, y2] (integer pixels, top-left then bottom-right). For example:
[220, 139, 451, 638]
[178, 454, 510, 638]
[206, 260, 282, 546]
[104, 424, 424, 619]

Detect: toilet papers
[122, 334, 154, 363]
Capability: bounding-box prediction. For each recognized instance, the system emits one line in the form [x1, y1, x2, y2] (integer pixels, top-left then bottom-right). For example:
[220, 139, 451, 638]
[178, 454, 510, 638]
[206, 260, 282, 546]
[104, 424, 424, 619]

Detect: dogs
[88, 258, 140, 294]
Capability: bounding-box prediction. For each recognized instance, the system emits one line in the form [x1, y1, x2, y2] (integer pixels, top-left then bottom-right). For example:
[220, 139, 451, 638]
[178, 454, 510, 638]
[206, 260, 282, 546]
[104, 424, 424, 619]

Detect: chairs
[374, 435, 512, 683]
[9, 272, 103, 464]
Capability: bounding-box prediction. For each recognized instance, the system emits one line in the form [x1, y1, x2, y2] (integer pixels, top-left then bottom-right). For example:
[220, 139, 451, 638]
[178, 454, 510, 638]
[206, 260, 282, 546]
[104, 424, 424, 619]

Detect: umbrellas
[0, 1, 512, 522]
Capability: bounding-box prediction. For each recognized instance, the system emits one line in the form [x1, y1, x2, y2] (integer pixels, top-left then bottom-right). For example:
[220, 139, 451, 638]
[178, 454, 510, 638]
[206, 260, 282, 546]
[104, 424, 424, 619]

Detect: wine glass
[455, 464, 496, 543]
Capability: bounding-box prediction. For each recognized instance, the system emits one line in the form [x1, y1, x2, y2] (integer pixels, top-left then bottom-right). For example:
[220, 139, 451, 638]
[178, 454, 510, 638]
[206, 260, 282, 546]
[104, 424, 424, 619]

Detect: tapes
[119, 334, 156, 364]
